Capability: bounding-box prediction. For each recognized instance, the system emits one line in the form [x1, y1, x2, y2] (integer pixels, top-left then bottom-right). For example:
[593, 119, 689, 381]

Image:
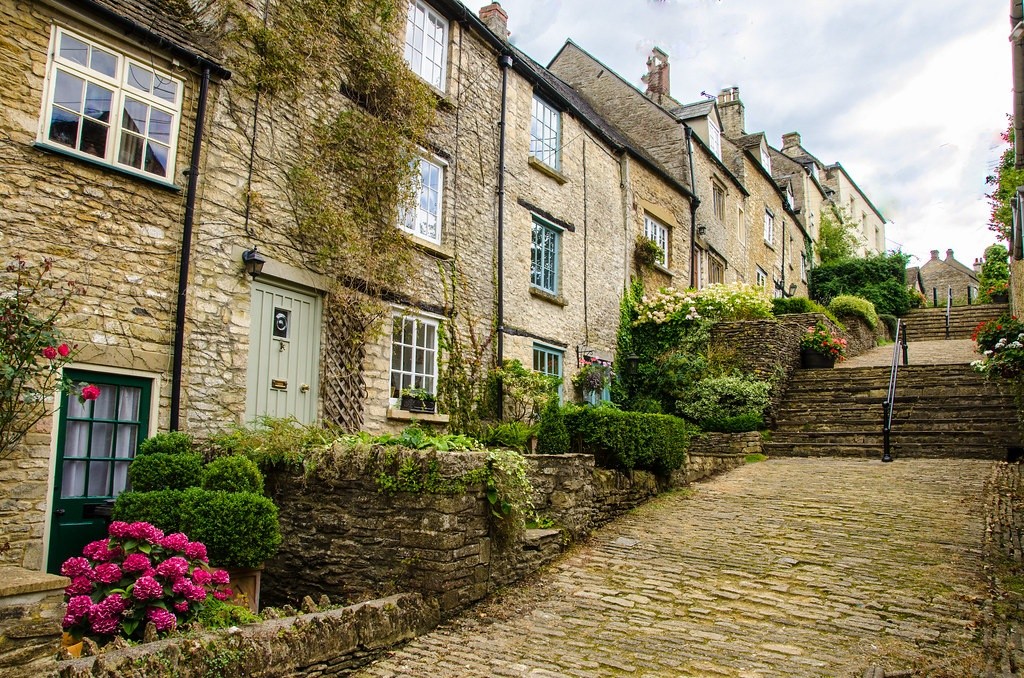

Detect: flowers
[802, 320, 847, 363]
[911, 292, 927, 303]
[988, 282, 1010, 297]
[571, 357, 618, 396]
[970, 315, 1024, 396]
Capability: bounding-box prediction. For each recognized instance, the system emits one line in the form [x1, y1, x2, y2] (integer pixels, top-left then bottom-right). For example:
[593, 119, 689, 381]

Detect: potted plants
[401, 386, 438, 414]
[112, 430, 283, 615]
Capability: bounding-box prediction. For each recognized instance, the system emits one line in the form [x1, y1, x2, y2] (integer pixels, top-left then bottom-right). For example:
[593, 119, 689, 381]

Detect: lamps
[697, 223, 706, 235]
[783, 283, 797, 296]
[242, 245, 266, 282]
[618, 351, 639, 376]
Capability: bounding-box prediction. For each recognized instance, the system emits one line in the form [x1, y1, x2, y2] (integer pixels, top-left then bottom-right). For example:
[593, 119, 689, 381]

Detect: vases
[985, 345, 1002, 358]
[1000, 364, 1017, 378]
[992, 295, 1009, 303]
[911, 300, 920, 307]
[803, 350, 835, 369]
